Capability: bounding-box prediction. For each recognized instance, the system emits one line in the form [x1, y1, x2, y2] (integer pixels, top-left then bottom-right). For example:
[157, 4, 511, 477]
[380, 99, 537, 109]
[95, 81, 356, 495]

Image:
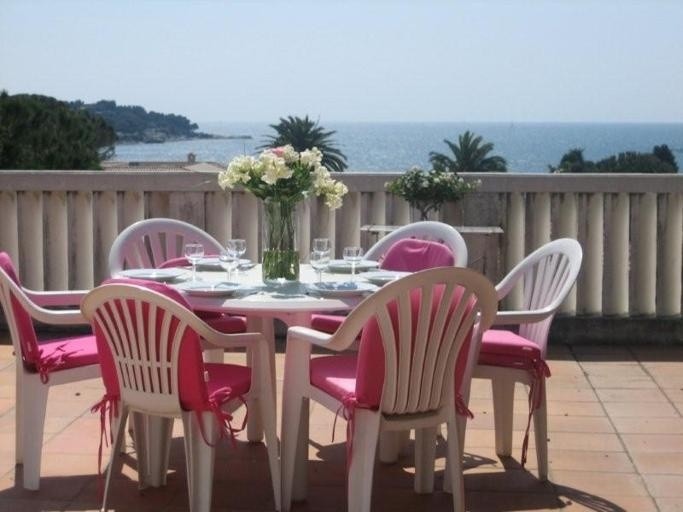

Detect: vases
[261, 200, 300, 287]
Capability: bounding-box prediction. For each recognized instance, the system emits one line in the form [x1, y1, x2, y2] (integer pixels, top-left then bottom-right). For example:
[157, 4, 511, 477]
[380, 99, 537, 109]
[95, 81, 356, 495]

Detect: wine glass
[313, 238, 332, 259]
[225, 239, 247, 282]
[232, 239, 248, 257]
[219, 251, 240, 282]
[309, 253, 330, 281]
[342, 247, 364, 275]
[184, 242, 205, 282]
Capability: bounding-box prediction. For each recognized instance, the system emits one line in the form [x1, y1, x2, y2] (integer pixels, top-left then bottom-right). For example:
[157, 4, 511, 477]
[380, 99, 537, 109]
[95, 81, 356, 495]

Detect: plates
[309, 281, 377, 296]
[327, 260, 379, 271]
[195, 258, 251, 268]
[359, 271, 413, 285]
[178, 286, 241, 297]
[117, 269, 185, 282]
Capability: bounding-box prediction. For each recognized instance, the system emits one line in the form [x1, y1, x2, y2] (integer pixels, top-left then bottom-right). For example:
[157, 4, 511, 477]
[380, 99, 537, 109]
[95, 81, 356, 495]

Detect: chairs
[362, 220, 469, 268]
[0, 253, 126, 491]
[80, 283, 282, 512]
[108, 218, 270, 448]
[400, 238, 583, 494]
[282, 265, 497, 512]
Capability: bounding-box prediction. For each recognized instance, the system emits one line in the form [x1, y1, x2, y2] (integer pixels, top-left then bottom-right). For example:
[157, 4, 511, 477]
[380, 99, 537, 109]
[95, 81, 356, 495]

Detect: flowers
[382, 166, 482, 224]
[218, 144, 349, 279]
